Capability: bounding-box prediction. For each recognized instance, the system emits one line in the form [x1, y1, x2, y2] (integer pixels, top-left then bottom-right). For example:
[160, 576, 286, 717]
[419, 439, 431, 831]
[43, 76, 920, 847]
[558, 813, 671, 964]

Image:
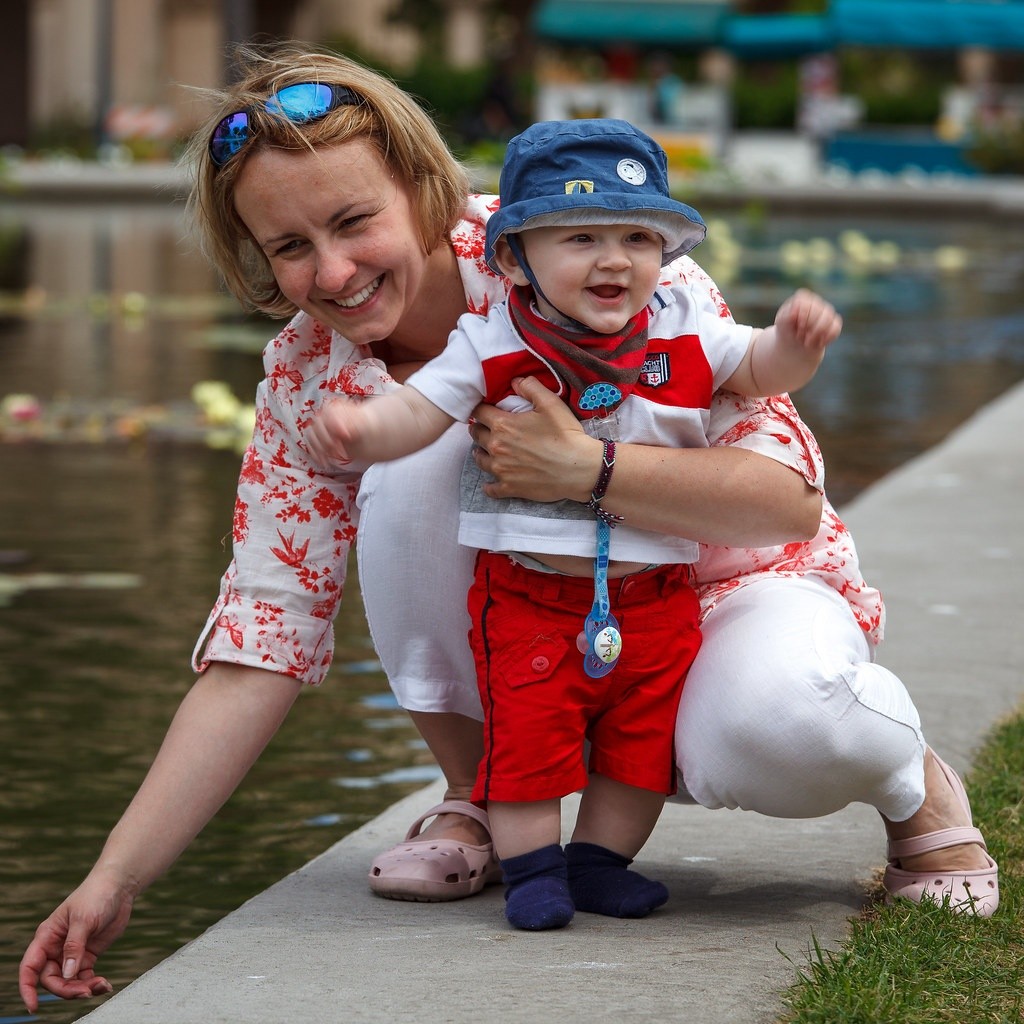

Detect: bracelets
[585, 437, 625, 529]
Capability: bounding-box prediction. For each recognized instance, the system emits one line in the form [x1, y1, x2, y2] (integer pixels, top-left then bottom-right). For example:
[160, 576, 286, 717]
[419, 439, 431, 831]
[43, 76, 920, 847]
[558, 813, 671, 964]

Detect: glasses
[207, 81, 373, 178]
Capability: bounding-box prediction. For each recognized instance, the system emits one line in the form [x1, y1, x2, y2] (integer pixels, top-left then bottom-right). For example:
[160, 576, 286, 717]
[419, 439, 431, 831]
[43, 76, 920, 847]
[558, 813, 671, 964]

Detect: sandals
[882, 743, 1000, 917]
[367, 799, 500, 903]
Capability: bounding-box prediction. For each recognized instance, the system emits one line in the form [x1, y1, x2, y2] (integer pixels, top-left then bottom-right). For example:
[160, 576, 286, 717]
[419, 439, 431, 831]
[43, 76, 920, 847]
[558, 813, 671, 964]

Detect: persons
[301, 119, 844, 931]
[21, 47, 1000, 1014]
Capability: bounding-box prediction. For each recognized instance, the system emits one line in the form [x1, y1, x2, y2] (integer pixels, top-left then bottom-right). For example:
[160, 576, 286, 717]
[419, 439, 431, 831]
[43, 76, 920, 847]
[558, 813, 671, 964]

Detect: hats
[484, 118, 709, 277]
